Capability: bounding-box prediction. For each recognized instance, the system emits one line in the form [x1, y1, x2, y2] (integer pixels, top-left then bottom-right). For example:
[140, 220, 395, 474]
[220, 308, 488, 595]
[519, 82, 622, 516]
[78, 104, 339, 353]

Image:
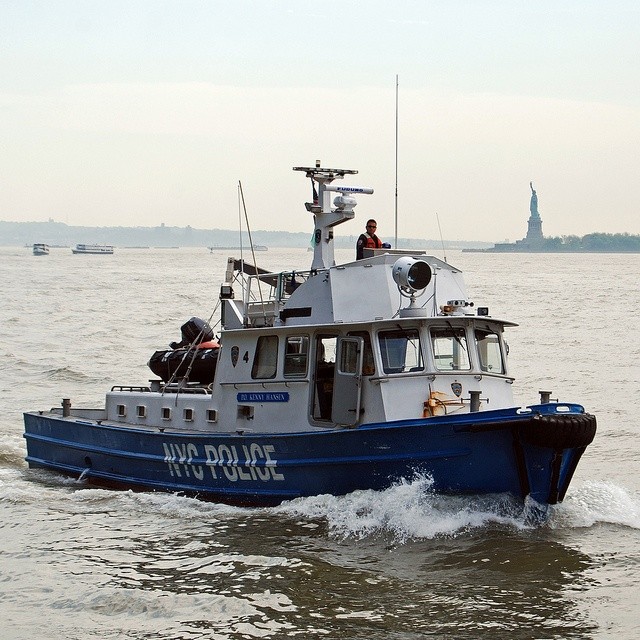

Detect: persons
[353, 218, 383, 260]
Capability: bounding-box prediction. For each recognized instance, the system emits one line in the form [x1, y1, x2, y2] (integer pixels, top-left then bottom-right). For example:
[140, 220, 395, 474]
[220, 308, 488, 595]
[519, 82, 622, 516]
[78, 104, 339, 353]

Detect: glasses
[367, 225, 376, 229]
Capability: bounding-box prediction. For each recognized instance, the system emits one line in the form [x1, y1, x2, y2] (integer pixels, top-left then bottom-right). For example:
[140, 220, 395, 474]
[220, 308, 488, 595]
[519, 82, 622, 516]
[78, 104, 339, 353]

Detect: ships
[72, 243, 114, 255]
[32, 243, 50, 255]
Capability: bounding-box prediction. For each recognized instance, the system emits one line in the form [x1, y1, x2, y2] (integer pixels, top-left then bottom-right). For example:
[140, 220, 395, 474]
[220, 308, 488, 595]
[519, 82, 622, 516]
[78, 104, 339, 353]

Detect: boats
[22, 72, 598, 510]
